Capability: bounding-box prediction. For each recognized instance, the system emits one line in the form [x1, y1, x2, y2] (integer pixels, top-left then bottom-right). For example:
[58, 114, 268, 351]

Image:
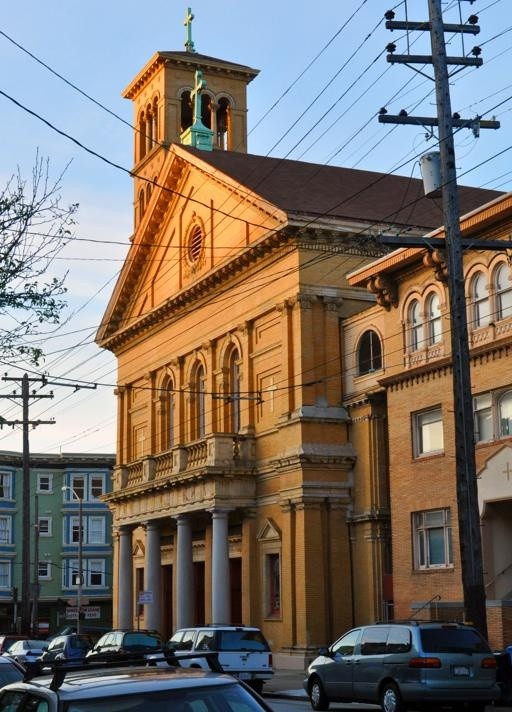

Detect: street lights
[60, 485, 83, 634]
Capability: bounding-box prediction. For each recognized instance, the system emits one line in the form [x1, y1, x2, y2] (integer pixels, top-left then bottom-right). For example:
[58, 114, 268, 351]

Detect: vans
[302, 619, 500, 712]
[0, 621, 278, 711]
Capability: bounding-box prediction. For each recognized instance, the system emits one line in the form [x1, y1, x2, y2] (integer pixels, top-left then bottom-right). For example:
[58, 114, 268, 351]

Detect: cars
[490, 644, 511, 708]
